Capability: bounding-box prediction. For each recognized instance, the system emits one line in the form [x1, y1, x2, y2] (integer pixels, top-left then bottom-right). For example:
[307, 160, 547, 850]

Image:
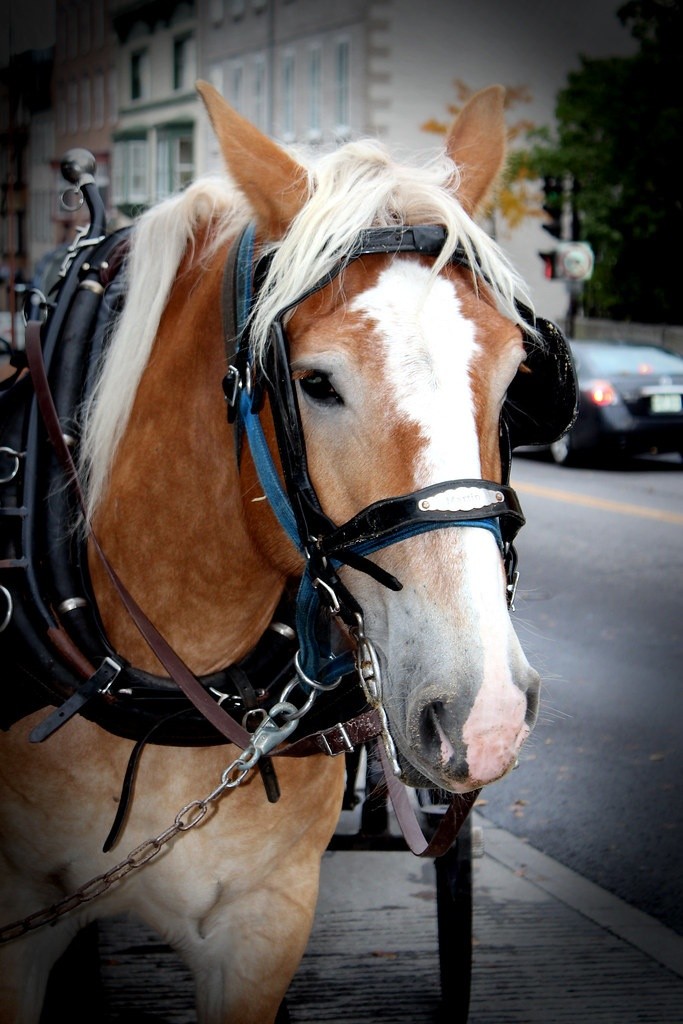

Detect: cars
[512, 337, 682, 466]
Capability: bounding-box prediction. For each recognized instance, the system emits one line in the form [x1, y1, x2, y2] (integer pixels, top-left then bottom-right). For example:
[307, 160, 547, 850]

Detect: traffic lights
[537, 252, 557, 282]
[541, 175, 562, 241]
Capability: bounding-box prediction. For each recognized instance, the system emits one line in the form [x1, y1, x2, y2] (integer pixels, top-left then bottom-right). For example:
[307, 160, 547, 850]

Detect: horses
[0, 75, 546, 1024]
